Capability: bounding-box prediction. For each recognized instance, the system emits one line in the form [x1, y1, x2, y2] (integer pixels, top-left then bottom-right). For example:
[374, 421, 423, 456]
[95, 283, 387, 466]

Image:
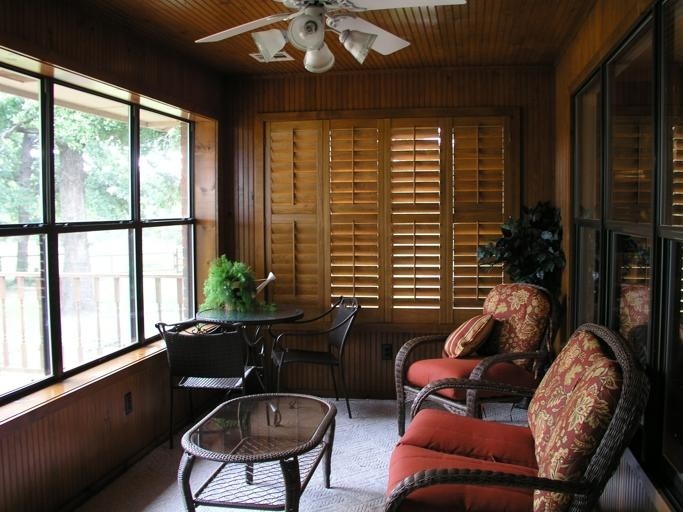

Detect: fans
[194, 0, 469, 75]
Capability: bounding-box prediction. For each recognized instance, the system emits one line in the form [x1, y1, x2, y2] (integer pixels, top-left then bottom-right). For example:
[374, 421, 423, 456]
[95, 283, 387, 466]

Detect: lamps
[250, 8, 378, 72]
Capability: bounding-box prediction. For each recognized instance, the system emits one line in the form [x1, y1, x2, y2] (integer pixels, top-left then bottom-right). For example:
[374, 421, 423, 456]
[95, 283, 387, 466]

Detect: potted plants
[197, 254, 277, 313]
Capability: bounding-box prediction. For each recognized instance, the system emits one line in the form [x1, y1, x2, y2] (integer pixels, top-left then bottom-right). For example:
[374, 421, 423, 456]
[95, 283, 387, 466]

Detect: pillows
[441, 315, 494, 359]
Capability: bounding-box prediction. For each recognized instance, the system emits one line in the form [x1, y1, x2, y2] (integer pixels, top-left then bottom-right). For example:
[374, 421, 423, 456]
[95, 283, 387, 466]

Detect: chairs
[384, 322, 640, 512]
[156, 294, 362, 448]
[395, 284, 567, 441]
